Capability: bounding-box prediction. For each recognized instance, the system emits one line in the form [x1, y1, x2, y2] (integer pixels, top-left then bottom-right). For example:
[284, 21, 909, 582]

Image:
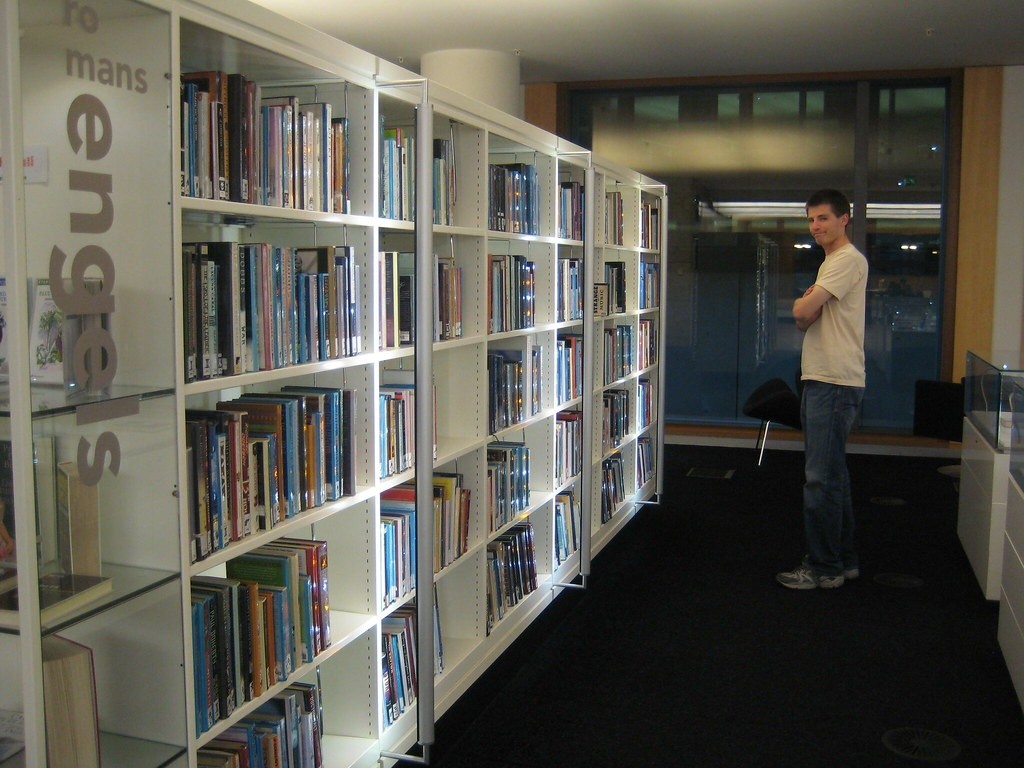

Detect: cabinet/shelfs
[0, 0, 668, 768]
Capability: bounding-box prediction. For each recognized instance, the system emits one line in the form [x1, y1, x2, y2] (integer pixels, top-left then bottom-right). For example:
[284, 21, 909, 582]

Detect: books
[0, 65, 661, 768]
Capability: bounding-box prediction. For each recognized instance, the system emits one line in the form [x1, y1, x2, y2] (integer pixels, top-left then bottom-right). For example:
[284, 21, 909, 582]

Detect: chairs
[913, 376, 965, 477]
[739, 379, 804, 467]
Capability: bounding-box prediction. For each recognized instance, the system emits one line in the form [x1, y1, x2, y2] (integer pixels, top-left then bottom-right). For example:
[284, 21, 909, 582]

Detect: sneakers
[777, 563, 846, 591]
[801, 553, 859, 579]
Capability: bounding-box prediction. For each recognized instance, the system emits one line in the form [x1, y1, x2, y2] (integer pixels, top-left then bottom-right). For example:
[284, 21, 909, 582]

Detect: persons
[771, 187, 870, 592]
